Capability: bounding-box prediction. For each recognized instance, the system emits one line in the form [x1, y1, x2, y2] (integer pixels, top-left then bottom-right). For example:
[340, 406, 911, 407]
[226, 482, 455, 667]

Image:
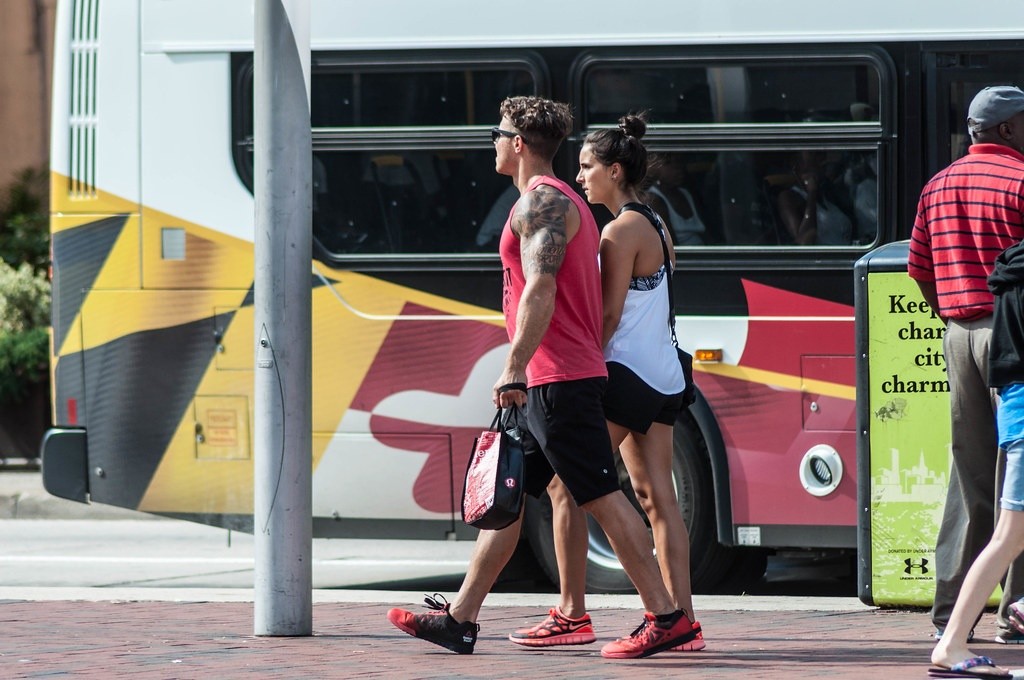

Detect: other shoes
[1008, 598, 1024, 634]
[935, 626, 974, 640]
[994, 635, 1024, 643]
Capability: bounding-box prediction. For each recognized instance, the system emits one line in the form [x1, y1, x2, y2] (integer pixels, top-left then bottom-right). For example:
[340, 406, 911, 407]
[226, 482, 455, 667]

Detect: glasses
[491, 127, 529, 146]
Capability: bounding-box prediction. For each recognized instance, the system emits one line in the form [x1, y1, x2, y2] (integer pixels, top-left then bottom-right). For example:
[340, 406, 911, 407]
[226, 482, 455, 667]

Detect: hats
[965, 86, 1024, 136]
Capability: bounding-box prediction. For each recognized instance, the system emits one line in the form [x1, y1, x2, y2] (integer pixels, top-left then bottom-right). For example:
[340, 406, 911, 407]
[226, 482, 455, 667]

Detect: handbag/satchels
[676, 346, 697, 409]
[461, 401, 527, 531]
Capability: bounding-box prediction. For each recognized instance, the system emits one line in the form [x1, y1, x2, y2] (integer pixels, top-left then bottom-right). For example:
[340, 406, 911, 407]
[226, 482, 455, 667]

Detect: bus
[41, 1, 1024, 597]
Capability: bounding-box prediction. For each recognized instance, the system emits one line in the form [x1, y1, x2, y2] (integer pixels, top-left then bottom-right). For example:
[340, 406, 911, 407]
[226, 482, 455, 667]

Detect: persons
[509, 122, 703, 651]
[640, 163, 704, 245]
[927, 243, 1022, 680]
[388, 96, 705, 661]
[907, 87, 1023, 642]
[843, 161, 879, 239]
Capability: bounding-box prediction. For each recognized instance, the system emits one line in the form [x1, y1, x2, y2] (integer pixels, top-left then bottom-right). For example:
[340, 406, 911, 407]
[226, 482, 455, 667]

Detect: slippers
[927, 655, 1013, 680]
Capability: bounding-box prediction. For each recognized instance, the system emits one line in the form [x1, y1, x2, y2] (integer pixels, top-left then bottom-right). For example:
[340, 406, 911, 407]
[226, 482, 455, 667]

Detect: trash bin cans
[854, 241, 1003, 608]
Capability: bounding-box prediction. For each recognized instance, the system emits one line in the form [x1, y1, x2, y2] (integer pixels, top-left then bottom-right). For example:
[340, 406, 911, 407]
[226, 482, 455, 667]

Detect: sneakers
[666, 620, 706, 650]
[600, 609, 696, 659]
[509, 605, 598, 650]
[386, 592, 480, 654]
